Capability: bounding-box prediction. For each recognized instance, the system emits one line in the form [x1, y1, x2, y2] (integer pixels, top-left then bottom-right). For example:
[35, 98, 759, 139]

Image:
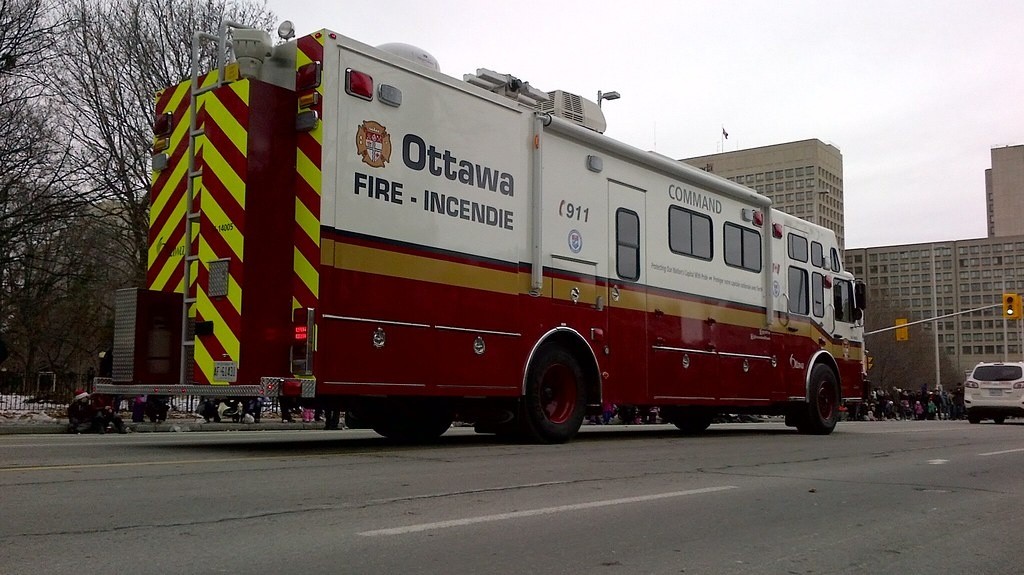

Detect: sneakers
[283, 417, 324, 422]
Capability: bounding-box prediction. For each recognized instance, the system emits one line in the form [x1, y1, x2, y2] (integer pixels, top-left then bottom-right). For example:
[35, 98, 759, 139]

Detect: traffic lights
[1003, 294, 1019, 319]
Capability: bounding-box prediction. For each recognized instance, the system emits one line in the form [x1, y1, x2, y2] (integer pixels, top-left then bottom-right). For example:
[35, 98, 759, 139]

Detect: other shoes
[323, 425, 343, 430]
[106, 425, 131, 434]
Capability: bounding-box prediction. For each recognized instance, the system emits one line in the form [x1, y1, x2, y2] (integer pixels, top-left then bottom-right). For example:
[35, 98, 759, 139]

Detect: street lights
[595, 88, 620, 107]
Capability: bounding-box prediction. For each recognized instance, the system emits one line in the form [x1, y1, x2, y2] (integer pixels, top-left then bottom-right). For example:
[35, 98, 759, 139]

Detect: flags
[723, 128, 728, 139]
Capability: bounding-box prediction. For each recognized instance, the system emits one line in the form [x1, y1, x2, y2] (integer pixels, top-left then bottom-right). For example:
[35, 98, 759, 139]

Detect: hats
[900, 400, 909, 407]
[75, 390, 89, 400]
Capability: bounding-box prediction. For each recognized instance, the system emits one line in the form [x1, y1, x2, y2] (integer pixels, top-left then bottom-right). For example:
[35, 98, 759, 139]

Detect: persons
[585, 403, 671, 425]
[67, 389, 132, 435]
[131, 395, 340, 429]
[858, 382, 967, 421]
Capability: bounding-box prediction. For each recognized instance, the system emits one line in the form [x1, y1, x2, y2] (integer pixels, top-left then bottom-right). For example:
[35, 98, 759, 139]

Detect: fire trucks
[91, 22, 868, 441]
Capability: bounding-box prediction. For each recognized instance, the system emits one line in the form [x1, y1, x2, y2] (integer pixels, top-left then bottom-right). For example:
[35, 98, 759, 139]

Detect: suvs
[963, 362, 1024, 424]
